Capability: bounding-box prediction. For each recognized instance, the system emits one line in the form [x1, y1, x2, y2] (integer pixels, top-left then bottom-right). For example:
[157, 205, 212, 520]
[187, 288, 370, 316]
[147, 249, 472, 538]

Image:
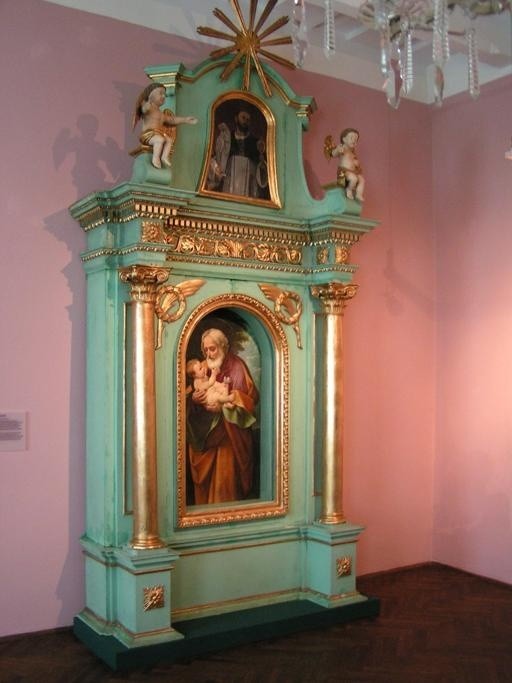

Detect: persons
[323, 127, 366, 203]
[207, 110, 269, 198]
[129, 83, 199, 169]
[187, 328, 259, 504]
[187, 358, 236, 414]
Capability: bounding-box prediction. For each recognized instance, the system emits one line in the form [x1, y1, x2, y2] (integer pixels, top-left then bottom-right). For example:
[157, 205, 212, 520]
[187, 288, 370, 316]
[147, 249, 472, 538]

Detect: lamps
[287, 0, 511, 112]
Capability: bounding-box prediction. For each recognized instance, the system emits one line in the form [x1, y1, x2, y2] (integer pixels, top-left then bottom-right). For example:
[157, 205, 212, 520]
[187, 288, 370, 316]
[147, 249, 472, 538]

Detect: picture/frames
[194, 87, 285, 211]
